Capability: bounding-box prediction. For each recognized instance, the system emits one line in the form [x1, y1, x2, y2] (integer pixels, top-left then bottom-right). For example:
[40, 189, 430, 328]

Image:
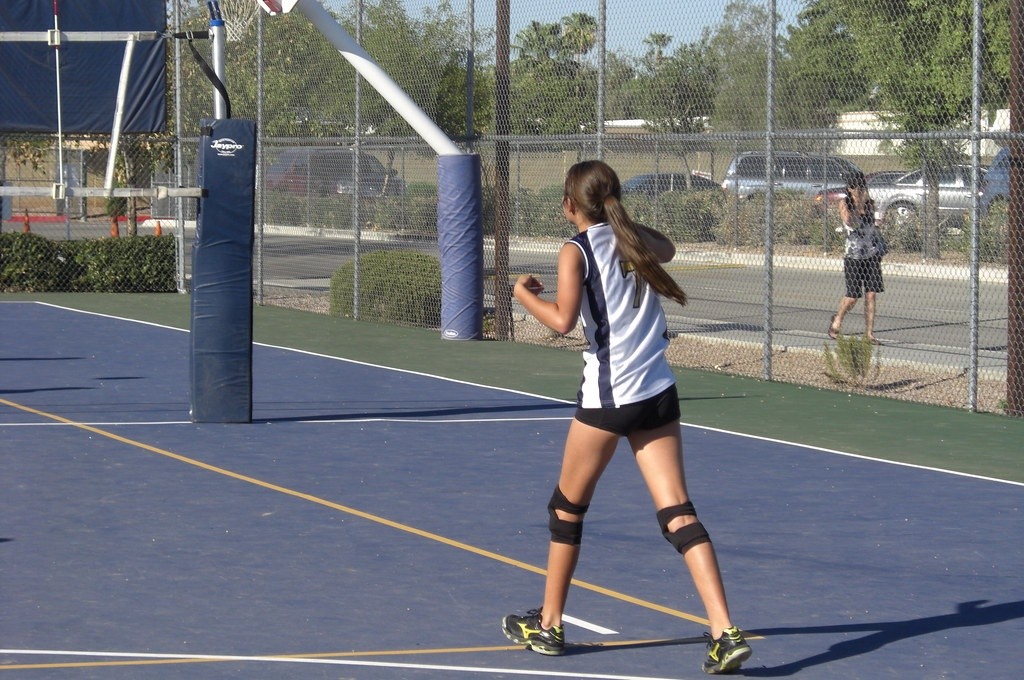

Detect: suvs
[982, 145, 1009, 241]
[722, 149, 863, 199]
[265, 147, 406, 202]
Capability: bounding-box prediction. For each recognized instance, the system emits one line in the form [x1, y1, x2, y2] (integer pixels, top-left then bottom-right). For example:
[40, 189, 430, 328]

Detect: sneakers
[701, 625, 753, 674]
[502, 606, 565, 656]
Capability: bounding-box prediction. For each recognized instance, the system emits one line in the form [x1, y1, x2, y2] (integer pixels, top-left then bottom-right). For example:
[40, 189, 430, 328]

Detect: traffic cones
[110, 209, 119, 237]
[155, 221, 162, 236]
[23, 209, 31, 233]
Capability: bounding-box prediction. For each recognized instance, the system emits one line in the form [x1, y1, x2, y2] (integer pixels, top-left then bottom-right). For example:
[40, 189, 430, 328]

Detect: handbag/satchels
[871, 226, 888, 258]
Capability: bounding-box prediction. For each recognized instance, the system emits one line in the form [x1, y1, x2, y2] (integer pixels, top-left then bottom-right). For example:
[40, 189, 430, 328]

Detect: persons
[829, 170, 886, 345]
[499, 159, 751, 676]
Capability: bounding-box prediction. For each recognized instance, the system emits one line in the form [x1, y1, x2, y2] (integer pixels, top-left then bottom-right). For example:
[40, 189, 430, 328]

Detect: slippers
[867, 337, 880, 345]
[828, 314, 840, 340]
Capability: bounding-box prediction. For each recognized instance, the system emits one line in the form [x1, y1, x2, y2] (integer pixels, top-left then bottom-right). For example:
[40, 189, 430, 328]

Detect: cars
[861, 163, 989, 229]
[815, 170, 908, 214]
[618, 173, 723, 197]
